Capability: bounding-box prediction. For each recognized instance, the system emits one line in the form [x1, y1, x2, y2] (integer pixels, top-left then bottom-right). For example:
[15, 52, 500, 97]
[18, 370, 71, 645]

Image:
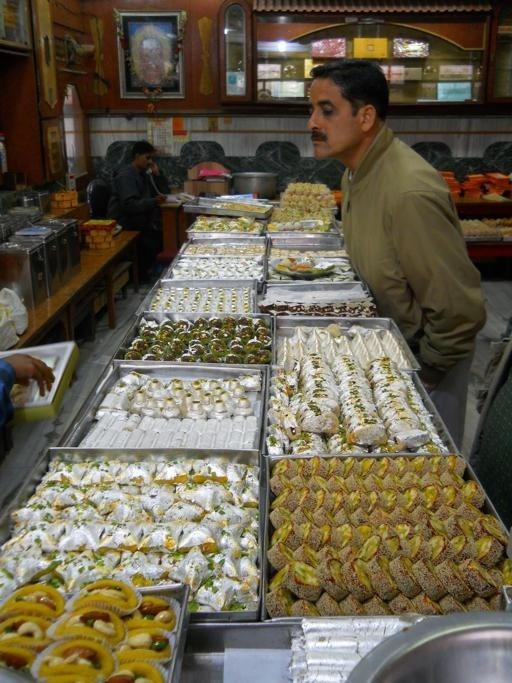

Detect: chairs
[462, 331, 510, 528]
[86, 141, 512, 234]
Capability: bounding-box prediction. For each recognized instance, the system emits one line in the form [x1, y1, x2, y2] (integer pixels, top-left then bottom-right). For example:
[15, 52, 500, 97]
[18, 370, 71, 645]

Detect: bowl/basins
[337, 616, 511, 683]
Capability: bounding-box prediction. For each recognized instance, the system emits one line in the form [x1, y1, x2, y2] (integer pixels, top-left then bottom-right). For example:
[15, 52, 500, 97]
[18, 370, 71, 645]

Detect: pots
[232, 172, 280, 199]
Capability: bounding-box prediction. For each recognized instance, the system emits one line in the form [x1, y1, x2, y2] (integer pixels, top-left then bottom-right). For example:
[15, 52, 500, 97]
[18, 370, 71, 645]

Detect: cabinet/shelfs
[0, 229, 140, 353]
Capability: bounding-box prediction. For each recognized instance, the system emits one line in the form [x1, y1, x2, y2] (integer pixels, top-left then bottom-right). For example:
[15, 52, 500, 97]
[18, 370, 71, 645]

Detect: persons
[105, 138, 171, 274]
[0, 350, 61, 473]
[303, 56, 489, 458]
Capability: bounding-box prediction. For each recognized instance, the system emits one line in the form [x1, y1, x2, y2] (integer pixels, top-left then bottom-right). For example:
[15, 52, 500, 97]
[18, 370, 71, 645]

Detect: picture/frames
[114, 10, 186, 101]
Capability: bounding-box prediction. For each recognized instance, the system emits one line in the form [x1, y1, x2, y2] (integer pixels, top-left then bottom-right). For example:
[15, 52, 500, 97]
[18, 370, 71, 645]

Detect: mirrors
[219, 1, 253, 104]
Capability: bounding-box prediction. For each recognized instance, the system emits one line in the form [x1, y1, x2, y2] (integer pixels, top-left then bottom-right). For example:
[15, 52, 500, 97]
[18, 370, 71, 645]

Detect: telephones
[146, 167, 152, 175]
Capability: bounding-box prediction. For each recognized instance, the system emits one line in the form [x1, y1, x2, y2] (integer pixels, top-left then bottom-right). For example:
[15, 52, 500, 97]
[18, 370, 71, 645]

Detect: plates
[273, 258, 335, 278]
